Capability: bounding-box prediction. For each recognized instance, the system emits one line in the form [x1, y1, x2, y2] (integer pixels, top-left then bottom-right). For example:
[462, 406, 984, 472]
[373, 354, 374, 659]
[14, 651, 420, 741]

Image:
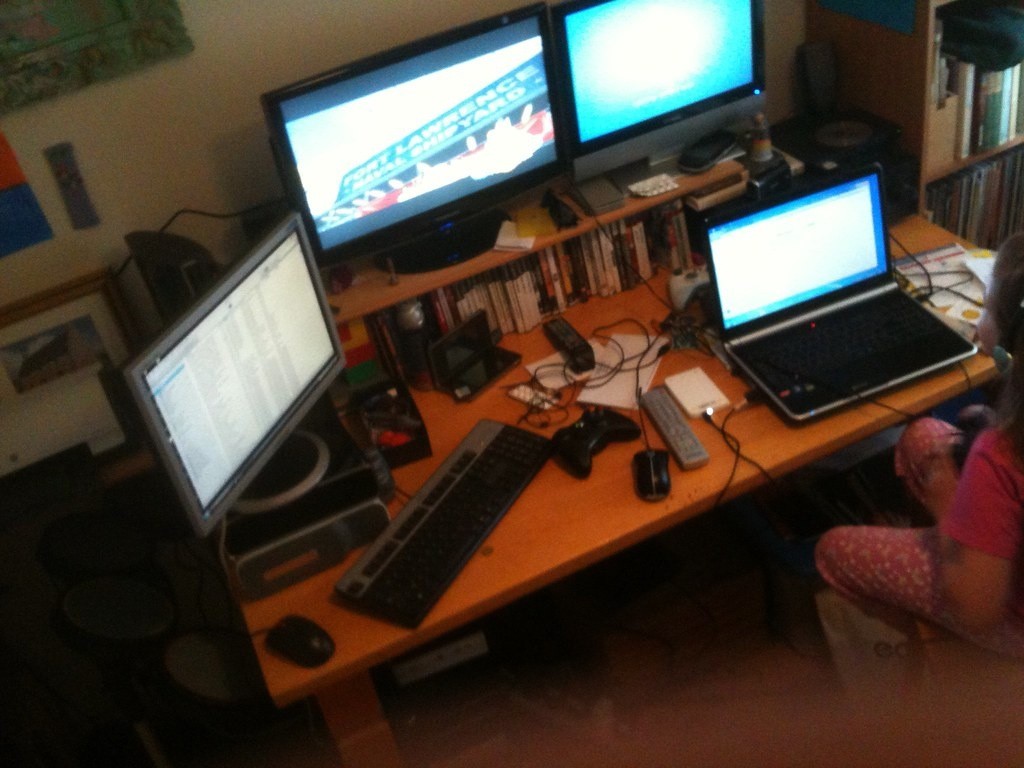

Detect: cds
[816, 122, 873, 147]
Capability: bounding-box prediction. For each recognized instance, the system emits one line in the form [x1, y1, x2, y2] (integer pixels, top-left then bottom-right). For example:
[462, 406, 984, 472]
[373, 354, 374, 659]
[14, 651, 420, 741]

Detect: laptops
[701, 159, 979, 426]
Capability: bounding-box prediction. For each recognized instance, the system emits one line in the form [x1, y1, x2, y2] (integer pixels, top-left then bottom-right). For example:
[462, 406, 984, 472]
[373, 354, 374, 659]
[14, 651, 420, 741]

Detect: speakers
[796, 41, 841, 115]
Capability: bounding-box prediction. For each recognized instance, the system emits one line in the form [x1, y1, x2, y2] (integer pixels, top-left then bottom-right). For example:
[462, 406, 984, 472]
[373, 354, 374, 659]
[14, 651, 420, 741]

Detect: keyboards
[326, 419, 557, 628]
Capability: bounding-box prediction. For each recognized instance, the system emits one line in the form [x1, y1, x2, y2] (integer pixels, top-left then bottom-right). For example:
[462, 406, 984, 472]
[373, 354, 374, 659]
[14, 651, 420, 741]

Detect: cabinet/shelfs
[802, 0, 1024, 253]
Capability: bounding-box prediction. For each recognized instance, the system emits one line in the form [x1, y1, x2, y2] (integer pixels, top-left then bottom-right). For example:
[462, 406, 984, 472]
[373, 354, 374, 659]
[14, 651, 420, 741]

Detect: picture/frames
[0, 0, 195, 118]
[0, 265, 158, 490]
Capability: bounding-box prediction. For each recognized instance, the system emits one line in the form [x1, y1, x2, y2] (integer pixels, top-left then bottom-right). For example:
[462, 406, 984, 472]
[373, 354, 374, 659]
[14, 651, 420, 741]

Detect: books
[923, 18, 1024, 249]
[421, 198, 693, 336]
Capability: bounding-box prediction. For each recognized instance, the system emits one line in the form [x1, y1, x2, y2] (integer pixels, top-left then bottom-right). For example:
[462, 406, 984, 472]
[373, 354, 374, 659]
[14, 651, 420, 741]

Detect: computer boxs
[214, 389, 391, 600]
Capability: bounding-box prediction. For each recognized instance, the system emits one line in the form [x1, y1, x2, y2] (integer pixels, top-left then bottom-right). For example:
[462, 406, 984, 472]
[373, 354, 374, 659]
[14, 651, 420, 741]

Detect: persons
[813, 232, 1024, 669]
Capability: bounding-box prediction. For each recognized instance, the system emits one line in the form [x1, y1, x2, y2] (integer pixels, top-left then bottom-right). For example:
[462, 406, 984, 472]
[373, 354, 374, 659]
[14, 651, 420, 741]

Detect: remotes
[543, 319, 596, 371]
[641, 388, 709, 471]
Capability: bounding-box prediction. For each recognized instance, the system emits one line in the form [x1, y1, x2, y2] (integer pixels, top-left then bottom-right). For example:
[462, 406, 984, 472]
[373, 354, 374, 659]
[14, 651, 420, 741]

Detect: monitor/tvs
[259, 0, 574, 273]
[546, 0, 770, 182]
[124, 211, 348, 542]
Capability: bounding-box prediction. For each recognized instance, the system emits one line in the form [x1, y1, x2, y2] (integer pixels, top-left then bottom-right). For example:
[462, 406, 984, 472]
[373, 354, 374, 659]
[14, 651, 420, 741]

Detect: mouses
[266, 616, 334, 669]
[632, 447, 670, 500]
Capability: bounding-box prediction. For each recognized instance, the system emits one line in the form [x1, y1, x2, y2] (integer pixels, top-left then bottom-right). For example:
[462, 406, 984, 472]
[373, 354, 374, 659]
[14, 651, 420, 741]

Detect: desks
[202, 129, 1009, 768]
